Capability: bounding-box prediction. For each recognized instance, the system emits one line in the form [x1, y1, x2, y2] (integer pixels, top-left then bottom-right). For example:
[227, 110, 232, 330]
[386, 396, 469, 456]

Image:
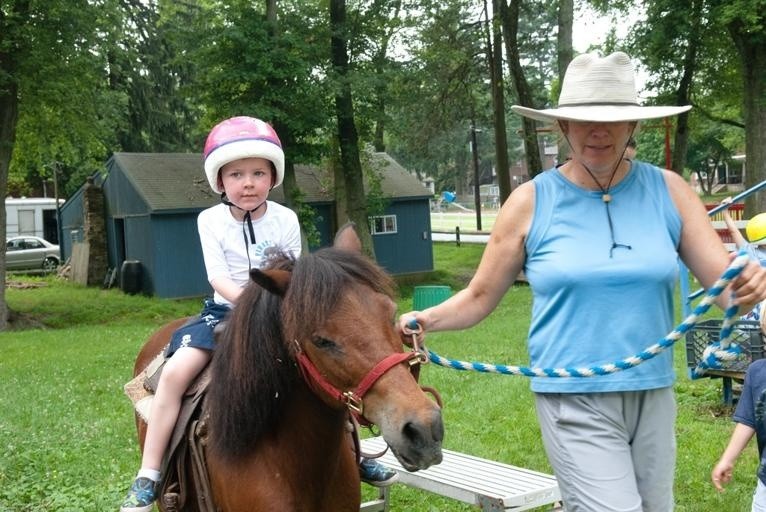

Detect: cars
[2, 233, 61, 272]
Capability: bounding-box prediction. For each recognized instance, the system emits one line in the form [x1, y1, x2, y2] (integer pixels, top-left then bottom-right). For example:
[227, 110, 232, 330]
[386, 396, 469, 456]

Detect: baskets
[686, 320, 765, 370]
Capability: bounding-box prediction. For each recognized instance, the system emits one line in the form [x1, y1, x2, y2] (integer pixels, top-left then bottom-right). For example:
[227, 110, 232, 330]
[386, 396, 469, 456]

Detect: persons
[396, 51, 766, 512]
[119, 116, 400, 512]
[623, 137, 636, 160]
[711, 300, 766, 512]
[720, 196, 766, 278]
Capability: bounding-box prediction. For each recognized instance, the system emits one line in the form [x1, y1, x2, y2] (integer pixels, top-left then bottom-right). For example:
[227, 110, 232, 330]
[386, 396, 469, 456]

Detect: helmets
[204, 117, 286, 194]
[746, 213, 766, 245]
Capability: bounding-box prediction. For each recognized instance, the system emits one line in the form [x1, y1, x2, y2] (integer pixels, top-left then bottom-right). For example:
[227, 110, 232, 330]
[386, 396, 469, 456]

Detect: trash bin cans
[413, 285, 453, 312]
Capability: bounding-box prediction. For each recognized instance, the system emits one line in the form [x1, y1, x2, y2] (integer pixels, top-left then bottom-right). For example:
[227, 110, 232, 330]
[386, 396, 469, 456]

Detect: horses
[132, 221, 445, 512]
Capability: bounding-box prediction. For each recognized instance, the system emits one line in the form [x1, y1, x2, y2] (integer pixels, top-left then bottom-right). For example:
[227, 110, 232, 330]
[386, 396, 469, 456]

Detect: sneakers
[120, 470, 159, 512]
[356, 458, 399, 487]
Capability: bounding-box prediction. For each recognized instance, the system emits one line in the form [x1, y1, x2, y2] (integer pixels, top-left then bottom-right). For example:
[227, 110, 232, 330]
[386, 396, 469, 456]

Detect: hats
[510, 52, 692, 122]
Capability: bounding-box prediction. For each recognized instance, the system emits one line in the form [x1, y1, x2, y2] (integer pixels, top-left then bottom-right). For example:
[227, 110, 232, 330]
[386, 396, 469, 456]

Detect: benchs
[358, 433, 562, 512]
